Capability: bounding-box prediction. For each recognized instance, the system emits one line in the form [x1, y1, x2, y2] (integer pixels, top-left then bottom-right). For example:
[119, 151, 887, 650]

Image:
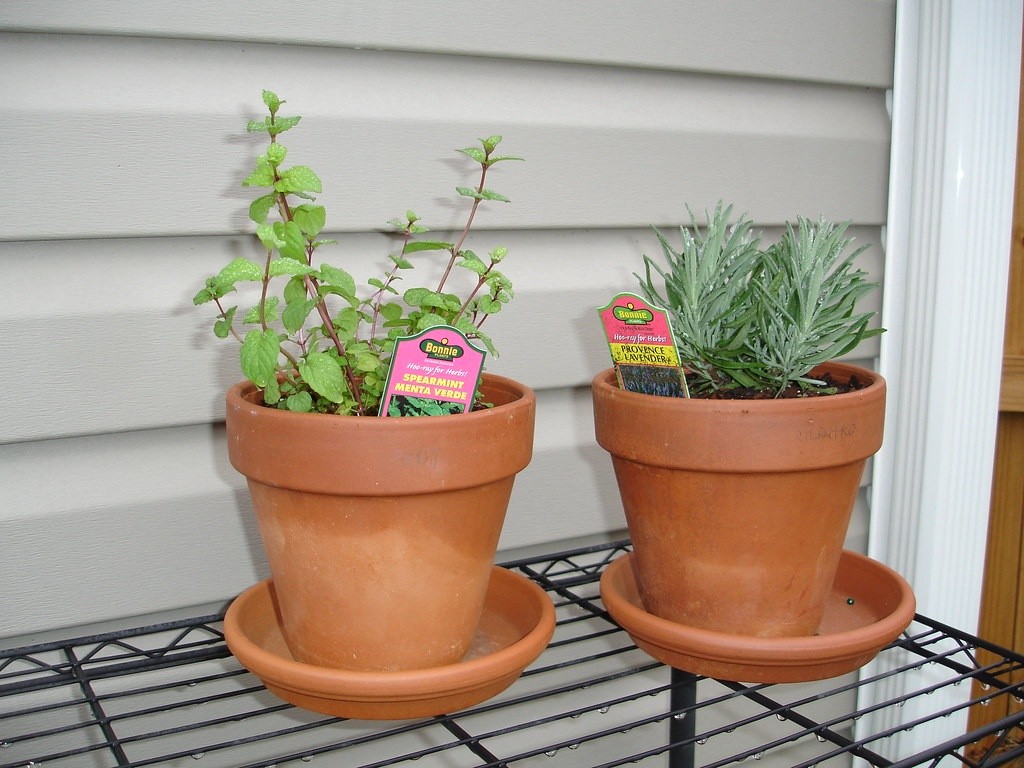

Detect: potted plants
[192, 91, 555, 720]
[590, 191, 922, 687]
